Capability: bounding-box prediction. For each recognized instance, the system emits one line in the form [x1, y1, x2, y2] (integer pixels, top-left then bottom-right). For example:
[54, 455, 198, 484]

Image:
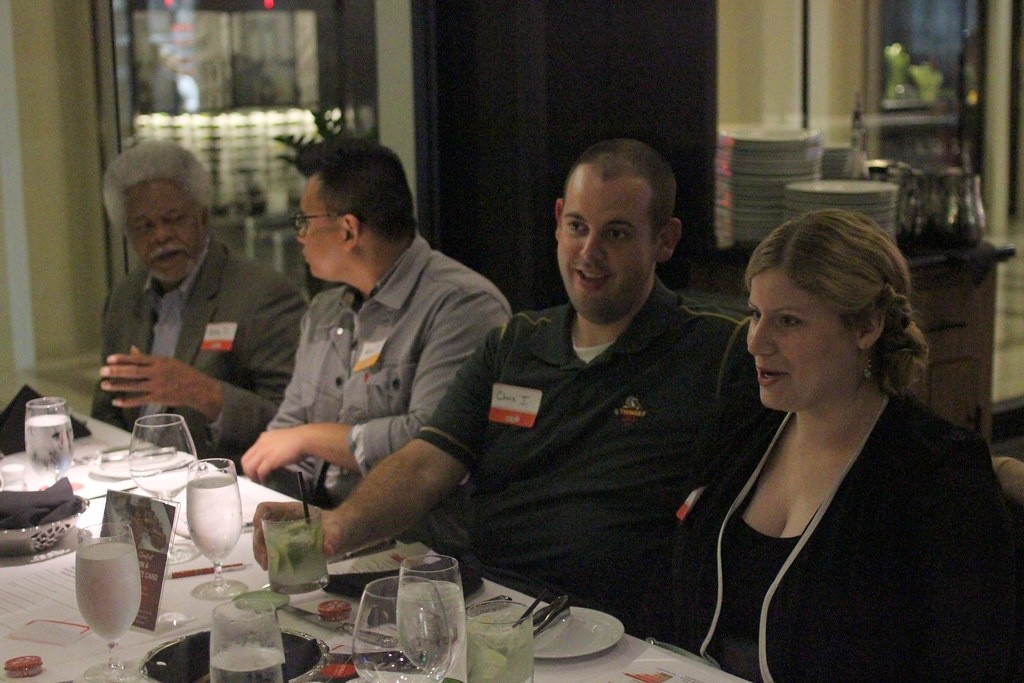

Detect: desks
[0, 412, 750, 683]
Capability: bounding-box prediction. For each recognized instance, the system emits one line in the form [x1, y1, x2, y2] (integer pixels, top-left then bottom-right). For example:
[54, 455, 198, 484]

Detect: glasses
[290, 211, 345, 237]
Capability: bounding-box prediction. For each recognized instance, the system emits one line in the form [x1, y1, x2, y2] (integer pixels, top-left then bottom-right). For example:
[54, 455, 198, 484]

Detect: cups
[352, 576, 449, 683]
[398, 554, 465, 683]
[209, 601, 283, 683]
[465, 601, 535, 683]
[260, 508, 330, 594]
[23, 397, 74, 498]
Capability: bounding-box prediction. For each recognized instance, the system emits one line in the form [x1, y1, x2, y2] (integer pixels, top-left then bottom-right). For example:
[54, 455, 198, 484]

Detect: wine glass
[186, 457, 249, 601]
[76, 523, 144, 683]
[128, 414, 201, 565]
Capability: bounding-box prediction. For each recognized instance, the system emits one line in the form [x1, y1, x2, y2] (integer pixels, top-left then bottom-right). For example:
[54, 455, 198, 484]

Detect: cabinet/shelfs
[705, 240, 1017, 442]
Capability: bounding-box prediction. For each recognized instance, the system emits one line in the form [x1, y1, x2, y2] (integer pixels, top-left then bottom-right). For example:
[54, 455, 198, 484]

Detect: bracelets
[990, 454, 1018, 475]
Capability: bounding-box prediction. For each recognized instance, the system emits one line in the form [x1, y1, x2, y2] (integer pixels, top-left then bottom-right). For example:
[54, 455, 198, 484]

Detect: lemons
[478, 647, 507, 680]
[274, 547, 304, 575]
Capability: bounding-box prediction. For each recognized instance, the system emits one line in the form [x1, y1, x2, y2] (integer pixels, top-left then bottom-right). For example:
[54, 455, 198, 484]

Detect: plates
[88, 448, 195, 479]
[534, 606, 624, 658]
[712, 124, 898, 252]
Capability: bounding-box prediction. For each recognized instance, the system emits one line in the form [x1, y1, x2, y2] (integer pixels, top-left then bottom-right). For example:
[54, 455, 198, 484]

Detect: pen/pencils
[170, 563, 245, 579]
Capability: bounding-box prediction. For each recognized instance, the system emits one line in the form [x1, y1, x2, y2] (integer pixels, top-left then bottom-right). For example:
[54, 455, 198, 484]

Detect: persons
[257, 139, 1024, 640]
[89, 141, 311, 477]
[241, 139, 512, 559]
[654, 208, 1024, 683]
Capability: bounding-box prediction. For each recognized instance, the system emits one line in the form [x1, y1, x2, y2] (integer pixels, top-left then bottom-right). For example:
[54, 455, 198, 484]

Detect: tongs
[533, 594, 569, 638]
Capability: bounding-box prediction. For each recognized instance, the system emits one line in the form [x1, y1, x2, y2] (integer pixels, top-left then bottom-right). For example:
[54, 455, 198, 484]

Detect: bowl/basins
[898, 170, 986, 254]
[0, 496, 90, 556]
